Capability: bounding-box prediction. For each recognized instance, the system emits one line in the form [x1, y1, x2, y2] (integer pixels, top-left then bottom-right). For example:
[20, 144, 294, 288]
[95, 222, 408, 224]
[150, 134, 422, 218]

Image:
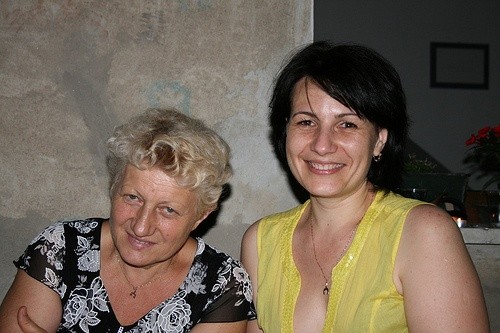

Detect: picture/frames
[428, 42, 490, 90]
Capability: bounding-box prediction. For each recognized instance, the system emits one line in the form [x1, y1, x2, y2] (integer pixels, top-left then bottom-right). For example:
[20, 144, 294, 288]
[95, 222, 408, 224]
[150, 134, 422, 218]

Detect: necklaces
[310, 193, 374, 294]
[113, 244, 173, 298]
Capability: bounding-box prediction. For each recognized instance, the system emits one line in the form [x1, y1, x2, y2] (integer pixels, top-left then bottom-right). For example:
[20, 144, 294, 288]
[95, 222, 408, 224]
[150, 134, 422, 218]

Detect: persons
[240, 39, 490, 333]
[0, 107, 258, 333]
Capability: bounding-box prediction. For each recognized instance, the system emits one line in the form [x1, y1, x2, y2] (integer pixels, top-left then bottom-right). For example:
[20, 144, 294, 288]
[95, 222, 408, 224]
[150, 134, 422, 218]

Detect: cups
[403, 188, 427, 200]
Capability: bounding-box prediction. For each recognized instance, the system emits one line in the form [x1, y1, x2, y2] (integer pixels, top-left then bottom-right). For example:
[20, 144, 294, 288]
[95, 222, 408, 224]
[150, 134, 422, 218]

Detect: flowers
[460, 124, 500, 192]
[400, 152, 438, 172]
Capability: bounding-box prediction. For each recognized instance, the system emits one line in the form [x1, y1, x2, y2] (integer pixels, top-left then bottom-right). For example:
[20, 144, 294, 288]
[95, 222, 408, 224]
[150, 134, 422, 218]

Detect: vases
[464, 189, 500, 225]
